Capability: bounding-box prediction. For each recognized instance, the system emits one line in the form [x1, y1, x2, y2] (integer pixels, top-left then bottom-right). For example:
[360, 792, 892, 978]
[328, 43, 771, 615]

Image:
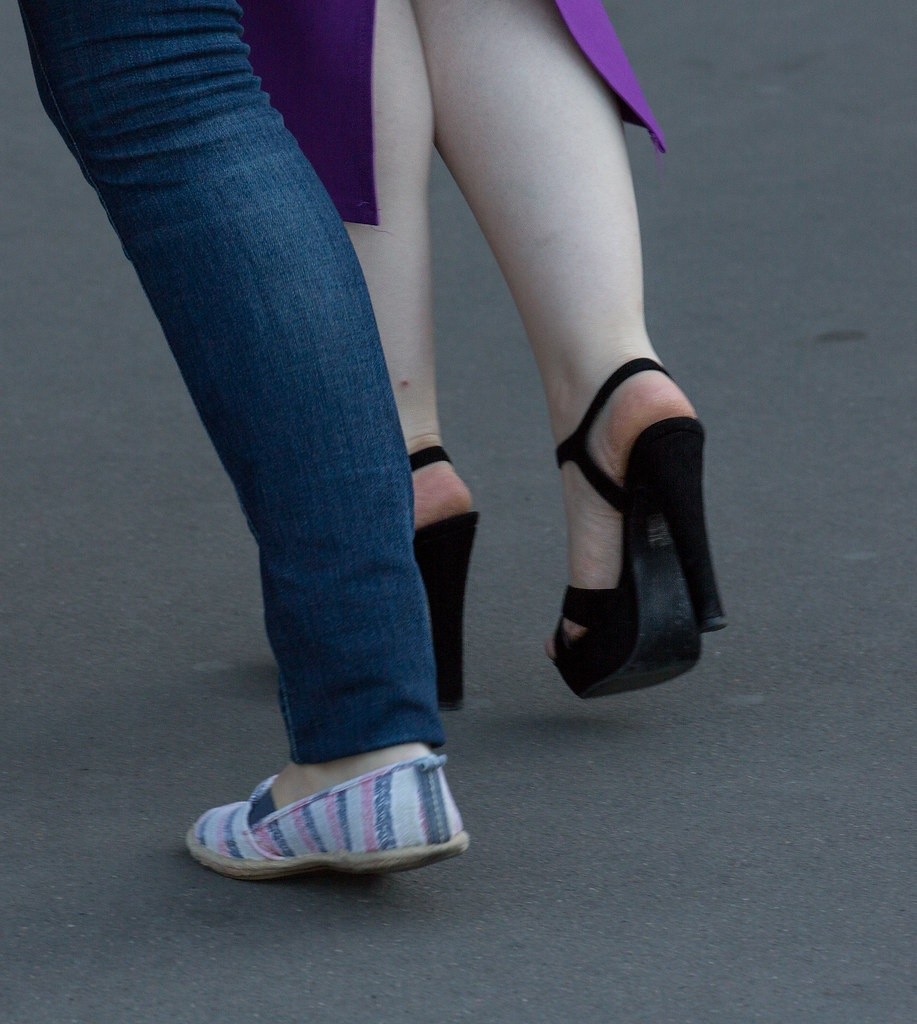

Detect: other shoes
[186, 752, 469, 881]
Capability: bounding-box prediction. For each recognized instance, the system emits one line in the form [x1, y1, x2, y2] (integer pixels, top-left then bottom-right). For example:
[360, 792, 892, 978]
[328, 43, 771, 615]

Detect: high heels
[405, 446, 484, 710]
[554, 356, 727, 700]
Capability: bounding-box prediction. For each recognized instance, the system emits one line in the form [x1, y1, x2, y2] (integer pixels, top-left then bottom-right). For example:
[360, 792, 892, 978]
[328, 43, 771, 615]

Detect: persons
[233, 0, 727, 708]
[15, 0, 473, 882]
